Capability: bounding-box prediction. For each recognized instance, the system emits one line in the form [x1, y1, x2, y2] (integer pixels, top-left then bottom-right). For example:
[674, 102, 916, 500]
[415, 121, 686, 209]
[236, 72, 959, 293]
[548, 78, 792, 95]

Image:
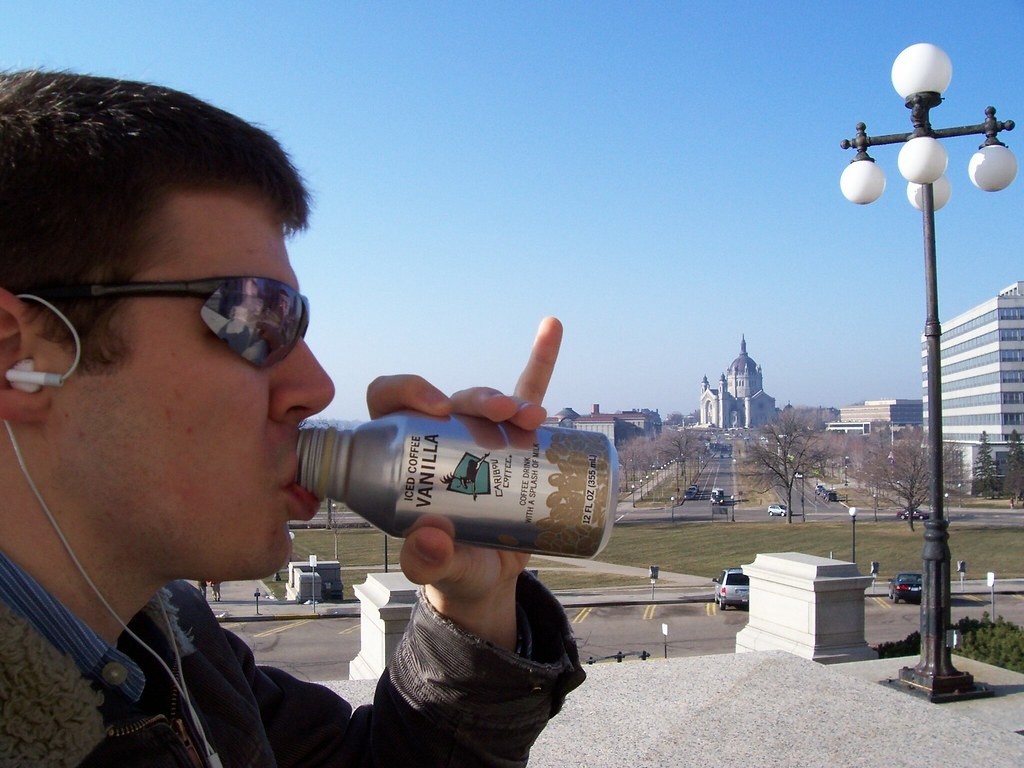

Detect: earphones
[4, 356, 66, 395]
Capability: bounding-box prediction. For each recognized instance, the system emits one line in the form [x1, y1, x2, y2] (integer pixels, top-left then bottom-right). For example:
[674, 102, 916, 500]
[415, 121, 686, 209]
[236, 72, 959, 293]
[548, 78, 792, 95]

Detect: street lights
[289, 531, 295, 562]
[660, 457, 679, 483]
[840, 40, 1019, 707]
[332, 503, 336, 528]
[873, 494, 877, 522]
[958, 483, 962, 508]
[640, 479, 644, 501]
[843, 455, 850, 486]
[848, 506, 857, 562]
[631, 484, 636, 507]
[944, 492, 950, 524]
[652, 472, 655, 492]
[645, 475, 649, 496]
[656, 470, 659, 486]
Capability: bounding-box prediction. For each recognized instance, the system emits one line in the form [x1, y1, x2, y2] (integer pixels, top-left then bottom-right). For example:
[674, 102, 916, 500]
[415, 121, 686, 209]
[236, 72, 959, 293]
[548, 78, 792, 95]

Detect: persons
[196, 581, 208, 600]
[1010, 497, 1014, 509]
[208, 580, 221, 602]
[2, 60, 591, 767]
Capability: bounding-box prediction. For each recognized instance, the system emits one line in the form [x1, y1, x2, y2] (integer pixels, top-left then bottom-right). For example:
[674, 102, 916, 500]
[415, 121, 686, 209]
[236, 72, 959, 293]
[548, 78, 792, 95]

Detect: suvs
[711, 567, 750, 611]
[767, 504, 793, 517]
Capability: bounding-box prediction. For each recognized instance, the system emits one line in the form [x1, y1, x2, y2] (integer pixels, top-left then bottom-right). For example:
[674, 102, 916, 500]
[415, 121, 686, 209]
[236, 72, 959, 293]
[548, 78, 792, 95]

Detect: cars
[815, 485, 838, 502]
[683, 483, 699, 500]
[887, 572, 922, 605]
[896, 506, 929, 521]
[795, 471, 803, 479]
[709, 487, 725, 506]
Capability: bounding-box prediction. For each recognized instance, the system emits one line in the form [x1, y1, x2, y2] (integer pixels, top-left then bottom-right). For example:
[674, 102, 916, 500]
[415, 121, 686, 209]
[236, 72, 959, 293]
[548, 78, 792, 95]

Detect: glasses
[13, 276, 308, 371]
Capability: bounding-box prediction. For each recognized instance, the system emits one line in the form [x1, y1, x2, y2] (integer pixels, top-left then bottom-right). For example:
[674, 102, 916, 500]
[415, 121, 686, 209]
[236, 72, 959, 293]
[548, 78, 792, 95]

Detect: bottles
[294, 410, 618, 560]
